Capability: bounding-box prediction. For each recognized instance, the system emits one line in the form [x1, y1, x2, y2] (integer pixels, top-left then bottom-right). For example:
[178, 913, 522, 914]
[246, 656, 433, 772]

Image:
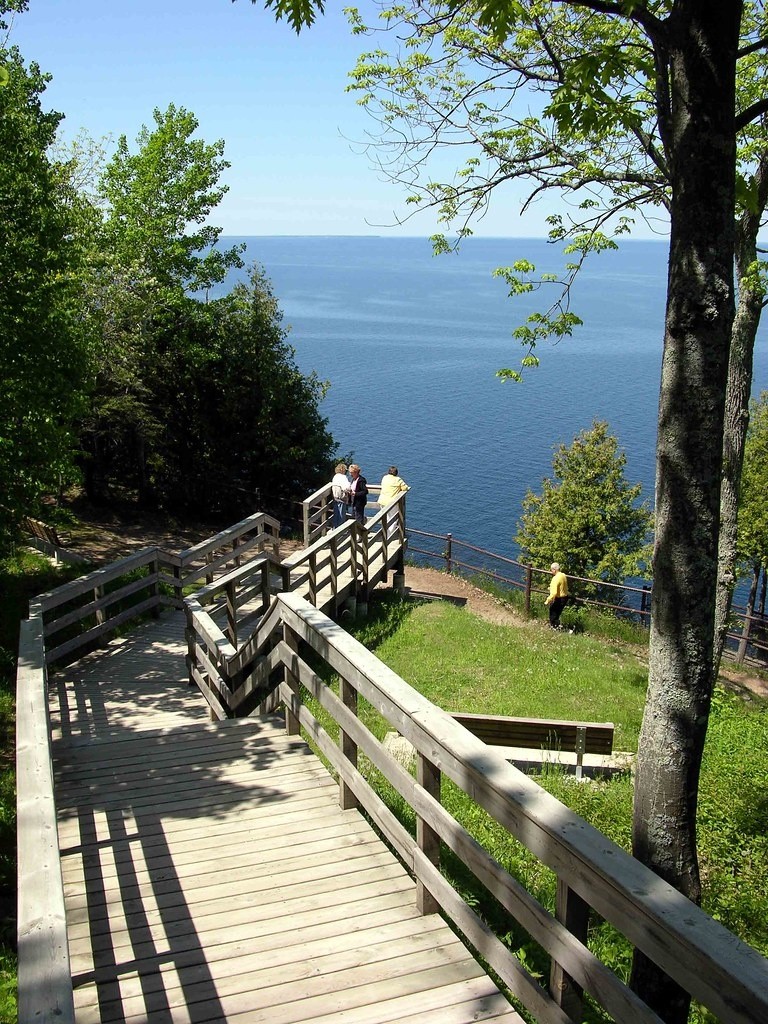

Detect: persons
[544, 563, 567, 628]
[377, 467, 409, 541]
[332, 464, 368, 544]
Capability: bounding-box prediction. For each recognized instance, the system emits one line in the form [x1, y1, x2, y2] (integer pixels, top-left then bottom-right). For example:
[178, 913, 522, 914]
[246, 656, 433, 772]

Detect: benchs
[0, 505, 78, 564]
[396, 711, 635, 783]
